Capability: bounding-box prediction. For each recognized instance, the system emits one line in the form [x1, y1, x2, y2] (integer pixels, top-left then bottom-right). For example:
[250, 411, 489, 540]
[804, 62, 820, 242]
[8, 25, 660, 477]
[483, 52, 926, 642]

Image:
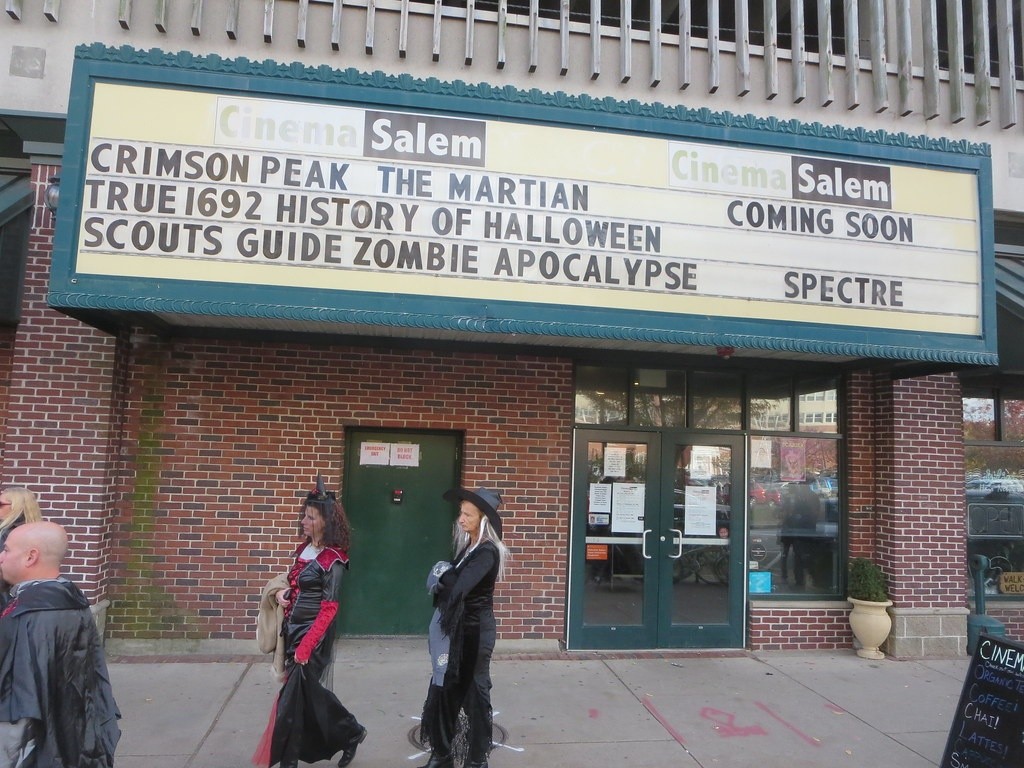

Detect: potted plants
[844, 556, 895, 660]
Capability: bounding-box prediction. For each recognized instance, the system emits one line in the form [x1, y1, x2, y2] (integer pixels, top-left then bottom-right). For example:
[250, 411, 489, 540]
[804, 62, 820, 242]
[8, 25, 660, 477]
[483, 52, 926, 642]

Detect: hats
[445, 486, 503, 539]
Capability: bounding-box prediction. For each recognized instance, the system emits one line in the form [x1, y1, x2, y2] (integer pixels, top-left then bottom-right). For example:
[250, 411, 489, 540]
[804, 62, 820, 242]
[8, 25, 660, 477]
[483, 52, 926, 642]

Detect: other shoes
[338, 727, 367, 767]
[463, 755, 487, 768]
[418, 751, 453, 768]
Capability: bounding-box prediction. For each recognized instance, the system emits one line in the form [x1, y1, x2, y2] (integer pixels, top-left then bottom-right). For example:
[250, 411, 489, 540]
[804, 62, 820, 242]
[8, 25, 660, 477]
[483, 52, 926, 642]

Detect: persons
[418, 485, 510, 768]
[252, 473, 367, 768]
[776, 472, 830, 586]
[718, 527, 729, 539]
[0, 487, 122, 768]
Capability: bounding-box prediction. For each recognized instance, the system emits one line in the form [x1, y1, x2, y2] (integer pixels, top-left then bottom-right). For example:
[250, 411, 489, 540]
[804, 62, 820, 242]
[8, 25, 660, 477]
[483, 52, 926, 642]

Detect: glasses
[0, 501, 11, 508]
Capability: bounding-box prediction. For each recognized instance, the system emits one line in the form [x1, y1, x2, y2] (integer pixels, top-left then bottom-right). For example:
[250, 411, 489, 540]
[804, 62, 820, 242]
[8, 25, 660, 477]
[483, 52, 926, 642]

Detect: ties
[0, 597, 17, 618]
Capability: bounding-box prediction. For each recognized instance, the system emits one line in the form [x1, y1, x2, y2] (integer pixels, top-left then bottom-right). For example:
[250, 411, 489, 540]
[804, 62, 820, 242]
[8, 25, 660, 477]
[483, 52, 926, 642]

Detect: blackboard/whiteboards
[931, 632, 1024, 768]
